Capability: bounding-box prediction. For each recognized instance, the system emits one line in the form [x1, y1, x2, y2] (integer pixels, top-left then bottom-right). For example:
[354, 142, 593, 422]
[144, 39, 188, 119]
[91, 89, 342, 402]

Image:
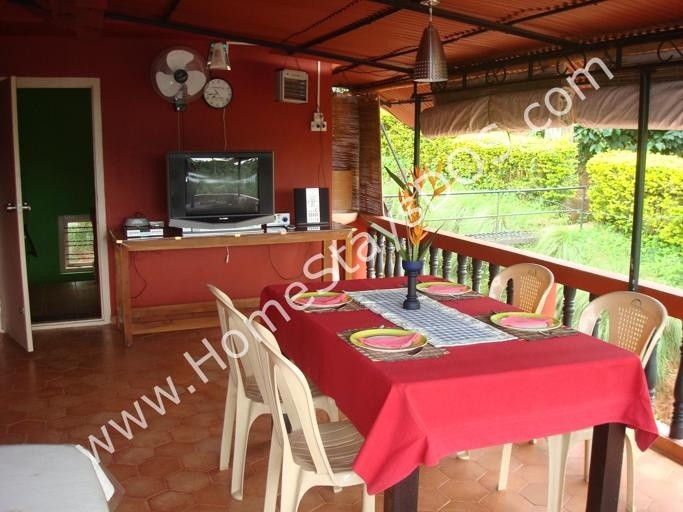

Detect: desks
[1, 445, 125, 510]
[109, 223, 357, 348]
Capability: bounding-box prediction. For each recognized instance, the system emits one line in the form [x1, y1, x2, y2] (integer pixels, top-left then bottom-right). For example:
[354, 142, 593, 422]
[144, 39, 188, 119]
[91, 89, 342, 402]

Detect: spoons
[537, 329, 577, 336]
[368, 347, 423, 360]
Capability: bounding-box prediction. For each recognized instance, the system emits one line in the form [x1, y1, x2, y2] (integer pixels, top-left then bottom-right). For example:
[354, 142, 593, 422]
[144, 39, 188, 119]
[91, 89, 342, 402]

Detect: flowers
[367, 158, 454, 263]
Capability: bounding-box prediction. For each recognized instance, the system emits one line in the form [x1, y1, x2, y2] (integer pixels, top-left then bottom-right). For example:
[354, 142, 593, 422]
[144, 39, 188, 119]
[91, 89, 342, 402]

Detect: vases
[401, 260, 424, 311]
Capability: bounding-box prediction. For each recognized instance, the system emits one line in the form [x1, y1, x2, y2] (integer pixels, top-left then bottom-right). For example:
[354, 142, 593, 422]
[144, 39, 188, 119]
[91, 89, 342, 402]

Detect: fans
[153, 47, 209, 110]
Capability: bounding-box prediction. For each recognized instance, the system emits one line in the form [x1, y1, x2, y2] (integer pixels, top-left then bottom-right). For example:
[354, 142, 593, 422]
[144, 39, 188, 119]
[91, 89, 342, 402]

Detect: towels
[424, 283, 470, 293]
[359, 332, 417, 348]
[294, 292, 346, 307]
[501, 316, 554, 326]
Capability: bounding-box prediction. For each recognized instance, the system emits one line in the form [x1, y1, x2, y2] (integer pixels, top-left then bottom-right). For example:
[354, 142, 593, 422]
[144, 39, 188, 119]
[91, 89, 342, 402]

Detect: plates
[291, 291, 353, 309]
[490, 311, 561, 332]
[350, 328, 428, 354]
[415, 281, 472, 297]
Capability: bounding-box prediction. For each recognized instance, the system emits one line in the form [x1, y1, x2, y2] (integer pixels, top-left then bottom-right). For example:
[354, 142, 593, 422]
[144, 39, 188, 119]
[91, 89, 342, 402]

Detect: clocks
[203, 78, 232, 109]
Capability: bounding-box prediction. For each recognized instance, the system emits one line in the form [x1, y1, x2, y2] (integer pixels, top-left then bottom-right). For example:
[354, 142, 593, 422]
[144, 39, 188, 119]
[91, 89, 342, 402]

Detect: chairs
[216, 296, 376, 512]
[456, 264, 554, 461]
[208, 283, 343, 501]
[497, 292, 668, 512]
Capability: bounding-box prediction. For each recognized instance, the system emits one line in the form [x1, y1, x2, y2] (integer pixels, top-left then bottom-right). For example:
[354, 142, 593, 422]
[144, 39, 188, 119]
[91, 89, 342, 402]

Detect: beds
[260, 275, 659, 511]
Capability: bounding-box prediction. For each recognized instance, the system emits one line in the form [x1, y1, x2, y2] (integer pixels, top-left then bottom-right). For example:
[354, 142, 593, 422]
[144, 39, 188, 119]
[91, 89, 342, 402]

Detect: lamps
[411, 0, 449, 82]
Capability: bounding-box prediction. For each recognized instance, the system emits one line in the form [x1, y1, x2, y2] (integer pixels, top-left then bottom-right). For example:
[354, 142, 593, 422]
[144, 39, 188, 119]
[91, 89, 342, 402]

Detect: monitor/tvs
[165, 150, 276, 236]
